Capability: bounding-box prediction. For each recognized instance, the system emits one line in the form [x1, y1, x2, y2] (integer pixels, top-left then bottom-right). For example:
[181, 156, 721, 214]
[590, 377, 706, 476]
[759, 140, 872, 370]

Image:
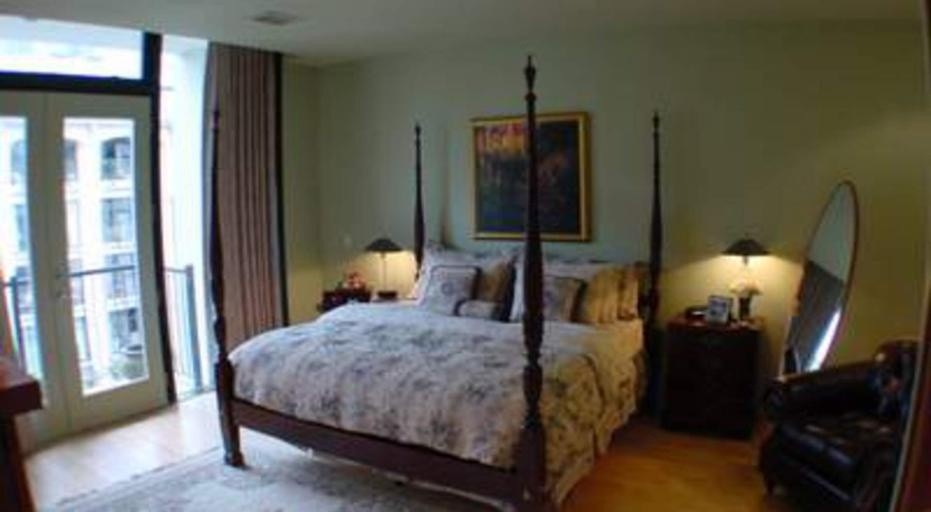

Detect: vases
[121, 342, 146, 379]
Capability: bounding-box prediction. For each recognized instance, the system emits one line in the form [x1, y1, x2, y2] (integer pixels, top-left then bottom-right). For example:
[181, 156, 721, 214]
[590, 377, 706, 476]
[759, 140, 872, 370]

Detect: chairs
[757, 339, 916, 511]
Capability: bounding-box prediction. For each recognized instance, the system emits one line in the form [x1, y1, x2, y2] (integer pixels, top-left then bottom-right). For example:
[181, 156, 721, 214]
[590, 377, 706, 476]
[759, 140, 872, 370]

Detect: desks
[0, 354, 49, 510]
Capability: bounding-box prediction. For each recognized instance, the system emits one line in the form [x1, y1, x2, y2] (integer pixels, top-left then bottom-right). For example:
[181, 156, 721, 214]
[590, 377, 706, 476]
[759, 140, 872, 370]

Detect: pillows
[506, 262, 580, 319]
[454, 299, 505, 320]
[409, 238, 518, 319]
[517, 248, 638, 323]
[417, 263, 480, 315]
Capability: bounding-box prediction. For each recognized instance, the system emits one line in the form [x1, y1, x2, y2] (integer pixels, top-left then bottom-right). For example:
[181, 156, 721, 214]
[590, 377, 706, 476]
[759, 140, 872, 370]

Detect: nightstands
[322, 289, 397, 312]
[664, 311, 763, 439]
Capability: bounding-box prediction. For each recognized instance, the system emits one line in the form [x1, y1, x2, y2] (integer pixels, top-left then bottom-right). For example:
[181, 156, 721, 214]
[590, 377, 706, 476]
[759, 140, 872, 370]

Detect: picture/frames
[465, 108, 591, 244]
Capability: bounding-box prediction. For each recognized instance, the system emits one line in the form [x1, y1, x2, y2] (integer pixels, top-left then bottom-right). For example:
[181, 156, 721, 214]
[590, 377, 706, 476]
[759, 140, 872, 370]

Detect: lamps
[362, 237, 405, 301]
[721, 239, 770, 318]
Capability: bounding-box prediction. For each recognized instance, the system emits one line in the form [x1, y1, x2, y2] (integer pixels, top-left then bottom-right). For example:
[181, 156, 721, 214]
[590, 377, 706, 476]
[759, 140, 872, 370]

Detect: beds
[209, 48, 663, 508]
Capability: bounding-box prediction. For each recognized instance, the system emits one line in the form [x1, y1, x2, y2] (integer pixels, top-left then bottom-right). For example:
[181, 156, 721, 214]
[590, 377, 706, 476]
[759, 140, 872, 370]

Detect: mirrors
[777, 178, 857, 375]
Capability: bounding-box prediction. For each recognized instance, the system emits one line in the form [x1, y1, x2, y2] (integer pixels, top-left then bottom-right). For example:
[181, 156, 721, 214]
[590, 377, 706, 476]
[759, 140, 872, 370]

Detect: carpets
[43, 438, 509, 510]
